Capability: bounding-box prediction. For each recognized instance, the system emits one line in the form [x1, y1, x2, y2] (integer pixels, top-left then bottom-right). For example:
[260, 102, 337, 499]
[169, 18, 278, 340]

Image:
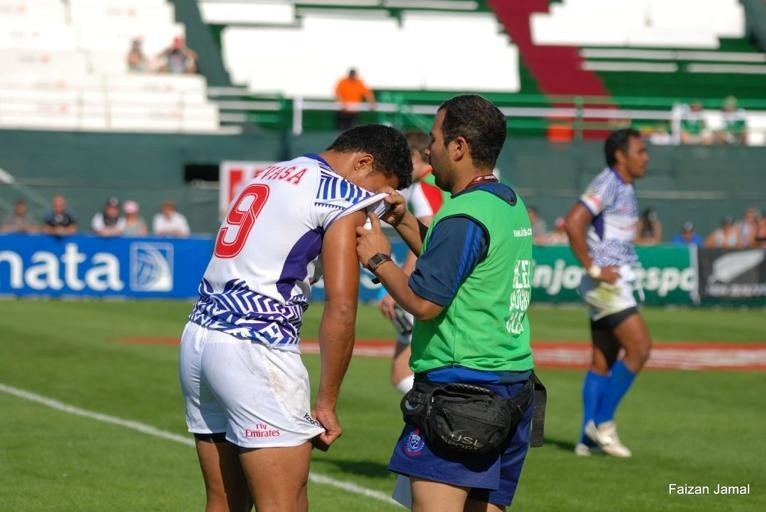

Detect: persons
[638, 206, 766, 247]
[527, 204, 546, 245]
[156, 35, 198, 74]
[0, 195, 191, 301]
[546, 216, 570, 244]
[713, 95, 748, 144]
[565, 126, 653, 459]
[667, 104, 710, 145]
[127, 35, 155, 74]
[179, 122, 415, 512]
[376, 128, 453, 399]
[333, 68, 377, 129]
[355, 94, 537, 511]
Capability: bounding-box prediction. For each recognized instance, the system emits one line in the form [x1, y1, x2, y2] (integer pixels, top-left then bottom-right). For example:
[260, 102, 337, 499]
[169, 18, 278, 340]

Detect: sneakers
[574, 415, 634, 460]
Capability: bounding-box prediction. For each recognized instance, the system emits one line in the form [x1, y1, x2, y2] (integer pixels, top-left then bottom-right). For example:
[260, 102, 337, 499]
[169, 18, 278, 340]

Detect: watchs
[367, 252, 392, 284]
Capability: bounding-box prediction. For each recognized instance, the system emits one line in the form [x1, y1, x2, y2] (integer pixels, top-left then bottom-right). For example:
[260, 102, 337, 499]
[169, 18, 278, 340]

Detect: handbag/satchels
[401, 374, 524, 470]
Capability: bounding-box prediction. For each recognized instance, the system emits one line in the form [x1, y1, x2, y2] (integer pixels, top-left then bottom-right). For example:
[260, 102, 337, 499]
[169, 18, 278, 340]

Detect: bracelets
[587, 264, 601, 279]
